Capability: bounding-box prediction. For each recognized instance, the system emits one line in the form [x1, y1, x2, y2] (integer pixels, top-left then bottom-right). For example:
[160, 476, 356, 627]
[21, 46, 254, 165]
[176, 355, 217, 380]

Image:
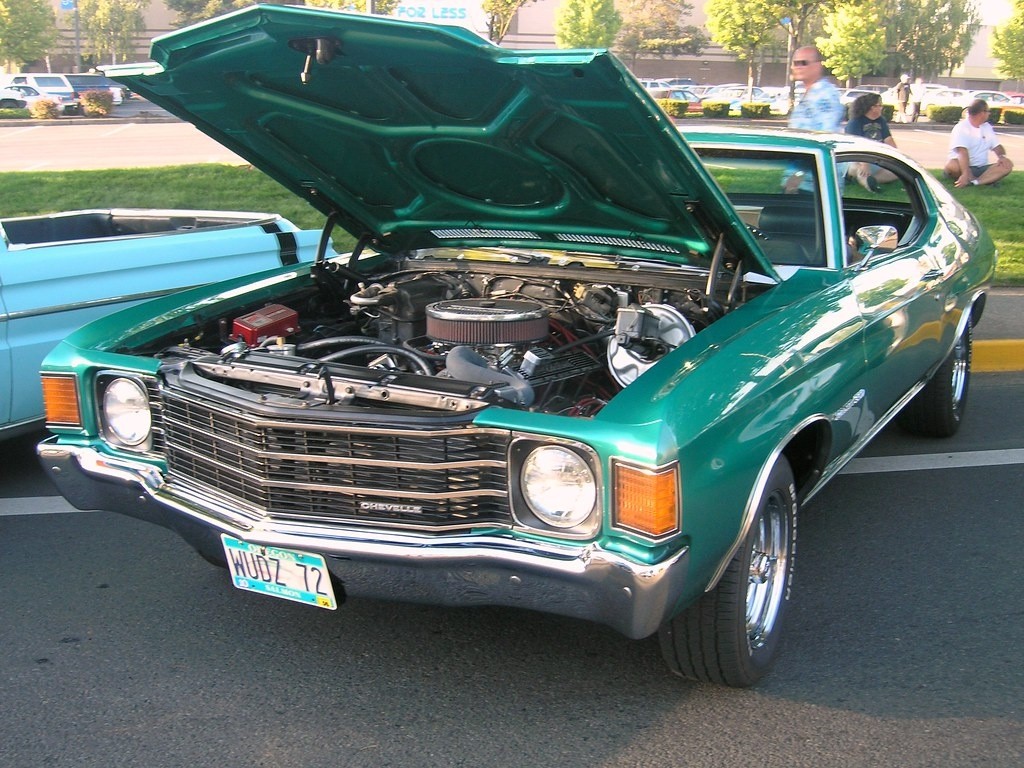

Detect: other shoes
[865, 176, 883, 194]
[988, 180, 1001, 188]
[967, 180, 973, 188]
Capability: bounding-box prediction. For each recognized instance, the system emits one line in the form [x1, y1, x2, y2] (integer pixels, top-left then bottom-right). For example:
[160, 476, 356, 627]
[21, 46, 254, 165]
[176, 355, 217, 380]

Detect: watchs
[998, 154, 1008, 159]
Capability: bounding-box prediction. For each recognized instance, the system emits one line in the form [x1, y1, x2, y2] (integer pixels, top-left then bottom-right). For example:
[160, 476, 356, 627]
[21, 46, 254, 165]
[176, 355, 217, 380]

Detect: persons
[945, 99, 1014, 188]
[775, 46, 844, 196]
[838, 93, 900, 193]
[895, 74, 927, 124]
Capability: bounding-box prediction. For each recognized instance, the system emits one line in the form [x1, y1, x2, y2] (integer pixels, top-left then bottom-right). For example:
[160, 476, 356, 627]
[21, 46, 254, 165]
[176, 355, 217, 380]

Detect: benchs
[732, 199, 913, 245]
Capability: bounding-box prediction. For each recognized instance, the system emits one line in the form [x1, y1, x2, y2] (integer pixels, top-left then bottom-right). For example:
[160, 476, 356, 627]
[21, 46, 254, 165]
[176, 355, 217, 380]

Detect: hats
[901, 74, 911, 80]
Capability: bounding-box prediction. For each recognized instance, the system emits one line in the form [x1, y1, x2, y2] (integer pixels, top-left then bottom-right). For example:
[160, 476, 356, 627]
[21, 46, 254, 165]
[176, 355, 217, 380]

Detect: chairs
[758, 205, 852, 267]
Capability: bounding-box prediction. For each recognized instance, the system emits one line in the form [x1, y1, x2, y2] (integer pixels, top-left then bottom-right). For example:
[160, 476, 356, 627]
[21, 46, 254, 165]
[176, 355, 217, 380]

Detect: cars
[640, 78, 1024, 112]
[34, 4, 997, 689]
[1, 73, 130, 107]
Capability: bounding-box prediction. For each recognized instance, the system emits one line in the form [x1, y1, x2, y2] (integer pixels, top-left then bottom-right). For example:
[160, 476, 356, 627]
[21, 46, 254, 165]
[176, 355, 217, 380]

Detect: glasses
[793, 61, 820, 66]
[982, 110, 990, 113]
[873, 103, 882, 107]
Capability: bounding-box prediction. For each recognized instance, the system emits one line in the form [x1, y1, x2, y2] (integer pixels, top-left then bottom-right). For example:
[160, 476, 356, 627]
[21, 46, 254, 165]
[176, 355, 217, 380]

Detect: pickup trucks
[0, 208, 339, 442]
